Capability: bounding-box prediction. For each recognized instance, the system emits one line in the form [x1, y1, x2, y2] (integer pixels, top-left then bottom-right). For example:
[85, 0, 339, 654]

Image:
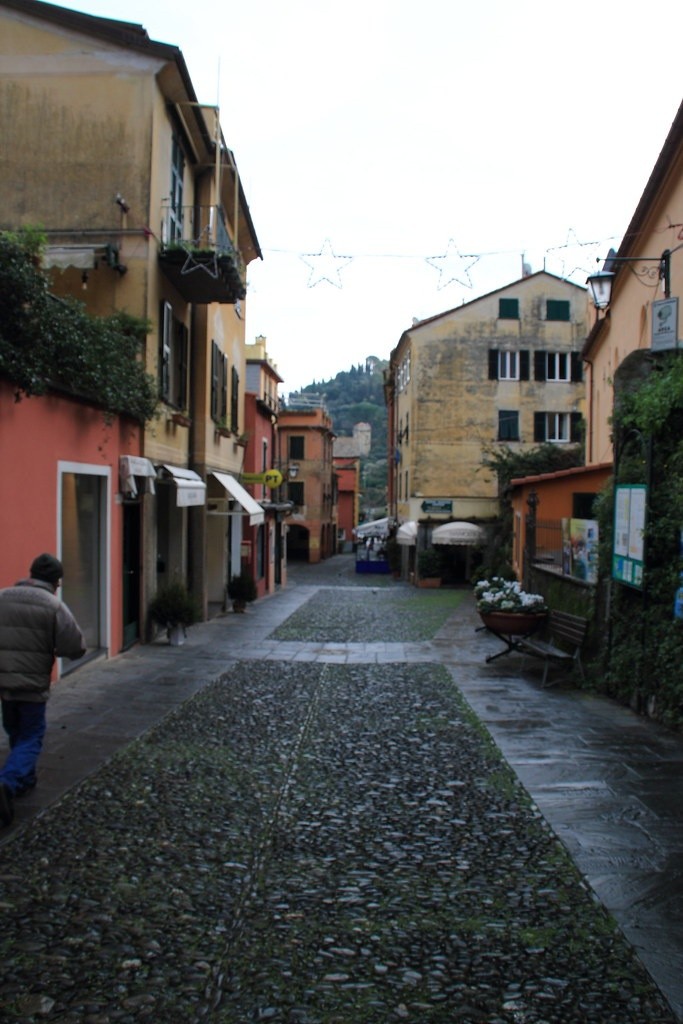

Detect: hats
[30, 553, 64, 583]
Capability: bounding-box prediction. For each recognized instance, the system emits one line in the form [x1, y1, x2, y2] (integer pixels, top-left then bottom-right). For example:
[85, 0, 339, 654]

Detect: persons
[0, 553, 87, 822]
[363, 536, 375, 551]
[377, 547, 386, 558]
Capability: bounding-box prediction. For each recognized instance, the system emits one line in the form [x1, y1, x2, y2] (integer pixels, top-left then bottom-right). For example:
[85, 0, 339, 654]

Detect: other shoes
[0, 782, 15, 828]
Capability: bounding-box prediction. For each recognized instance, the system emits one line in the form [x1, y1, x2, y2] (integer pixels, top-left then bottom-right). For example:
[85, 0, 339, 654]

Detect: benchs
[519, 610, 589, 689]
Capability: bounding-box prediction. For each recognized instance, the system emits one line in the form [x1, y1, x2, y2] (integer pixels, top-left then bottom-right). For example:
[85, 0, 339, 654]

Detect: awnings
[352, 517, 389, 538]
[432, 522, 485, 546]
[211, 470, 265, 526]
[163, 463, 206, 507]
[397, 521, 417, 545]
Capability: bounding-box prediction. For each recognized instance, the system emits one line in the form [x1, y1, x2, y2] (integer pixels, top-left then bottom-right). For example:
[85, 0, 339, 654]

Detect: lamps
[586, 249, 670, 313]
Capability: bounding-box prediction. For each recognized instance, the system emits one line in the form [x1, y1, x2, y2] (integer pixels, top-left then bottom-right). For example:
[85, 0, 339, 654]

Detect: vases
[172, 413, 192, 426]
[476, 609, 545, 633]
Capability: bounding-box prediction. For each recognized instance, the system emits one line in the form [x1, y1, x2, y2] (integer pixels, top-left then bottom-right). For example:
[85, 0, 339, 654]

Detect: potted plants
[150, 586, 205, 647]
[418, 549, 442, 587]
[228, 570, 258, 613]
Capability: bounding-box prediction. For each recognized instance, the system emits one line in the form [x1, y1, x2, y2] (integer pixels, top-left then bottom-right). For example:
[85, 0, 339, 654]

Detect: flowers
[474, 577, 549, 615]
